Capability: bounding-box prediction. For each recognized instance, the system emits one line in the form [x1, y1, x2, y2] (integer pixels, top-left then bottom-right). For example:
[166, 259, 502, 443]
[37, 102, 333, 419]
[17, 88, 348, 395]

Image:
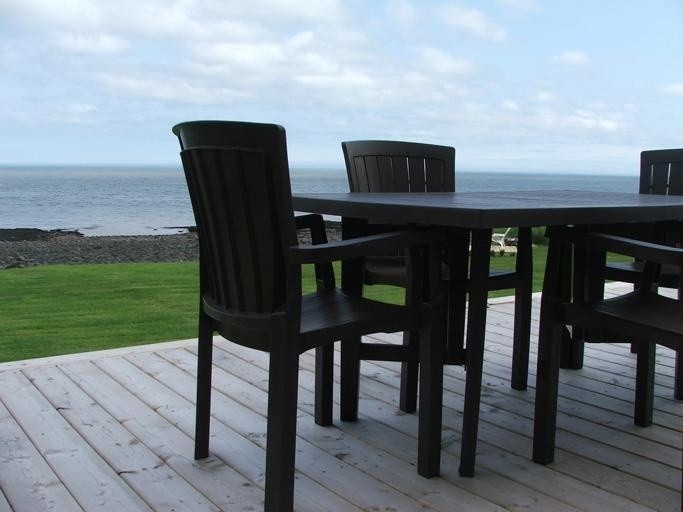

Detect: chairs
[339, 139, 532, 411]
[171, 120, 427, 512]
[573, 150, 682, 400]
[530, 234, 681, 466]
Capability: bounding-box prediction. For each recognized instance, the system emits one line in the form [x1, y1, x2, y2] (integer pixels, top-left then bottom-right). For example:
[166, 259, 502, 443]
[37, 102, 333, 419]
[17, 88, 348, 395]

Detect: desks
[281, 189, 682, 479]
